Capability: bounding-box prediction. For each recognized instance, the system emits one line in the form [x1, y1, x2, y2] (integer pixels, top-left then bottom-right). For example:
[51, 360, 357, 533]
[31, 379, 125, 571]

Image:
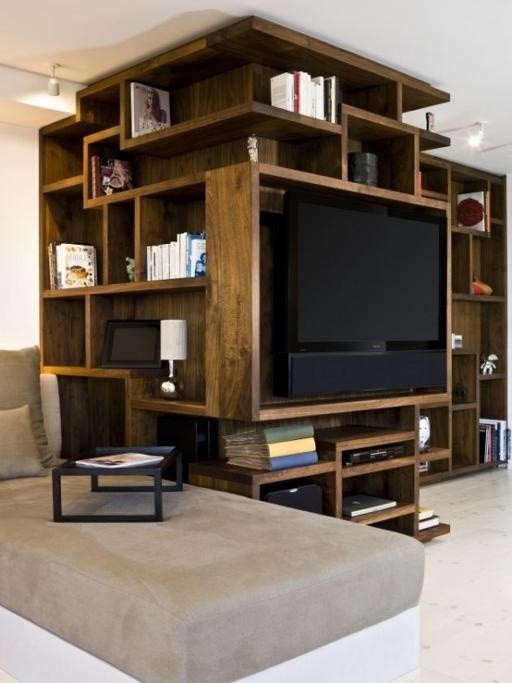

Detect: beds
[0, 344, 427, 683]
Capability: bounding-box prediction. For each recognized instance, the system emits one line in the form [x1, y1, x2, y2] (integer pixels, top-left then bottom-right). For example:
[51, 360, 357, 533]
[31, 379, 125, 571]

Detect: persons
[139, 88, 168, 131]
[418, 414, 431, 448]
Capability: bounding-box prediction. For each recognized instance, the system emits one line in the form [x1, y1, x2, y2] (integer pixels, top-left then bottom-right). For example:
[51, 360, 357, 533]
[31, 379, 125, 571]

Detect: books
[146, 226, 210, 284]
[456, 191, 485, 232]
[416, 516, 442, 531]
[417, 506, 433, 521]
[227, 451, 319, 471]
[342, 495, 397, 517]
[221, 423, 314, 443]
[269, 67, 339, 124]
[129, 81, 172, 140]
[75, 452, 163, 470]
[225, 436, 319, 457]
[45, 238, 99, 290]
[90, 154, 135, 200]
[479, 418, 507, 464]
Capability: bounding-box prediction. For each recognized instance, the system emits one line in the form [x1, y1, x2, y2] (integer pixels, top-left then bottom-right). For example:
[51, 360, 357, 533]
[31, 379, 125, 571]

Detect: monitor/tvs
[276, 185, 447, 398]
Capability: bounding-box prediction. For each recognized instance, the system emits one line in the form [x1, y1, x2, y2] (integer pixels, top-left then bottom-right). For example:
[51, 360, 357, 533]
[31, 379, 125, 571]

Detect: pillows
[9, 338, 67, 479]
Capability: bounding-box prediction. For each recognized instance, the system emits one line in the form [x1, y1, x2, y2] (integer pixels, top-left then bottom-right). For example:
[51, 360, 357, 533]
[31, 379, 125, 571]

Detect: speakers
[265, 484, 326, 515]
